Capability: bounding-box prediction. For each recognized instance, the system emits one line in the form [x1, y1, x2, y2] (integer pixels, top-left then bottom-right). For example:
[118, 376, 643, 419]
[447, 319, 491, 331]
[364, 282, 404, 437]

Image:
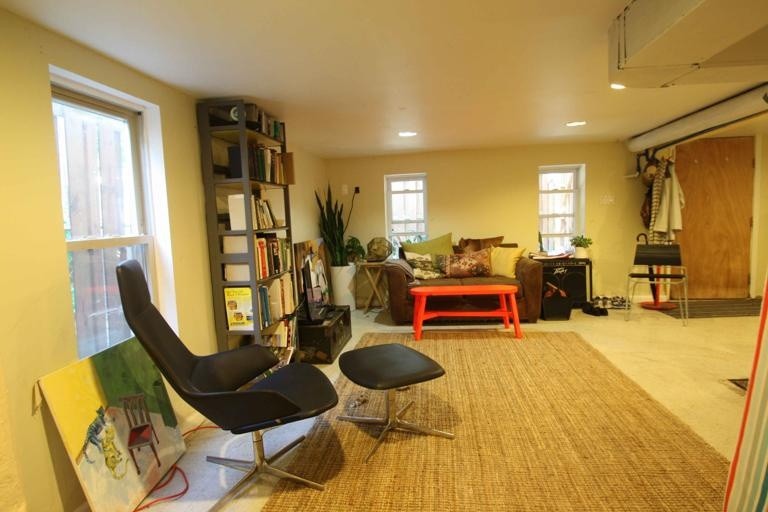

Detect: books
[210, 104, 298, 368]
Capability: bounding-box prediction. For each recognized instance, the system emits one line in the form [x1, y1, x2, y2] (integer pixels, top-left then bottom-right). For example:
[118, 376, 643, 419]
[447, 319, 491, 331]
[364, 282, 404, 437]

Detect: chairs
[625, 239, 689, 326]
[115, 259, 338, 512]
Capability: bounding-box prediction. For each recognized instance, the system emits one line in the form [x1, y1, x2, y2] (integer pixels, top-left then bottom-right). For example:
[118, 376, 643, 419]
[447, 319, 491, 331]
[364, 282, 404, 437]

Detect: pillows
[459, 236, 504, 254]
[404, 252, 445, 280]
[399, 232, 454, 269]
[436, 248, 490, 278]
[490, 244, 526, 278]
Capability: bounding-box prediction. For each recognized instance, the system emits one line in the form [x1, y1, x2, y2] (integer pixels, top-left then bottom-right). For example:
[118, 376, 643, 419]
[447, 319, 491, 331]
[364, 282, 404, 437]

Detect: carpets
[260, 333, 730, 511]
[658, 295, 762, 319]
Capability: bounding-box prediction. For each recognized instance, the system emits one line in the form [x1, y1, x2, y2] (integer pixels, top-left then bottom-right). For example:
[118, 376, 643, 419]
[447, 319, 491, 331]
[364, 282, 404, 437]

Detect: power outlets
[355, 186, 359, 193]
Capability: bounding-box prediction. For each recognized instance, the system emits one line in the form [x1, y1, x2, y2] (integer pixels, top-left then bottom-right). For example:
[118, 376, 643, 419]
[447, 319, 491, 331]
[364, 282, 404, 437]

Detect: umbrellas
[636, 233, 656, 301]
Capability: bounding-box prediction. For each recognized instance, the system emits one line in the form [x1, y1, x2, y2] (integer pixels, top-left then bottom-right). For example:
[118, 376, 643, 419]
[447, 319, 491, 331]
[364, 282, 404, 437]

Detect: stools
[337, 343, 455, 462]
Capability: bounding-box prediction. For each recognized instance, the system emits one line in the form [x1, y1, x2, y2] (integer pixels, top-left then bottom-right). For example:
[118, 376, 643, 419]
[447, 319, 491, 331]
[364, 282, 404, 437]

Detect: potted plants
[570, 234, 593, 258]
[313, 179, 357, 312]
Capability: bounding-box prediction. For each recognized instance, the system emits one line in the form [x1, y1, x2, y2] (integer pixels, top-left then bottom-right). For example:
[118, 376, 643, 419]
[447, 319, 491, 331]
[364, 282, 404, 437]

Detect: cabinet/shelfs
[195, 100, 300, 365]
[295, 306, 352, 364]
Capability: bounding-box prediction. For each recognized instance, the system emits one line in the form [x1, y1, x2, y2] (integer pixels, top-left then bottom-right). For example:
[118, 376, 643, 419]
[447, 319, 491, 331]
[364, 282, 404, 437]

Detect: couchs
[385, 244, 543, 323]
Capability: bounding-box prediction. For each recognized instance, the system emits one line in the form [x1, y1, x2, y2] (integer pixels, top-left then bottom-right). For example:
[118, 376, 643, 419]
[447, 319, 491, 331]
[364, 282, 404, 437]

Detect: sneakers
[583, 296, 631, 316]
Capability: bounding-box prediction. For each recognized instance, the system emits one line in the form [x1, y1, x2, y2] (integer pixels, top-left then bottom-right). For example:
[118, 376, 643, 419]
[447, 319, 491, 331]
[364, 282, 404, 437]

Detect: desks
[354, 262, 385, 314]
